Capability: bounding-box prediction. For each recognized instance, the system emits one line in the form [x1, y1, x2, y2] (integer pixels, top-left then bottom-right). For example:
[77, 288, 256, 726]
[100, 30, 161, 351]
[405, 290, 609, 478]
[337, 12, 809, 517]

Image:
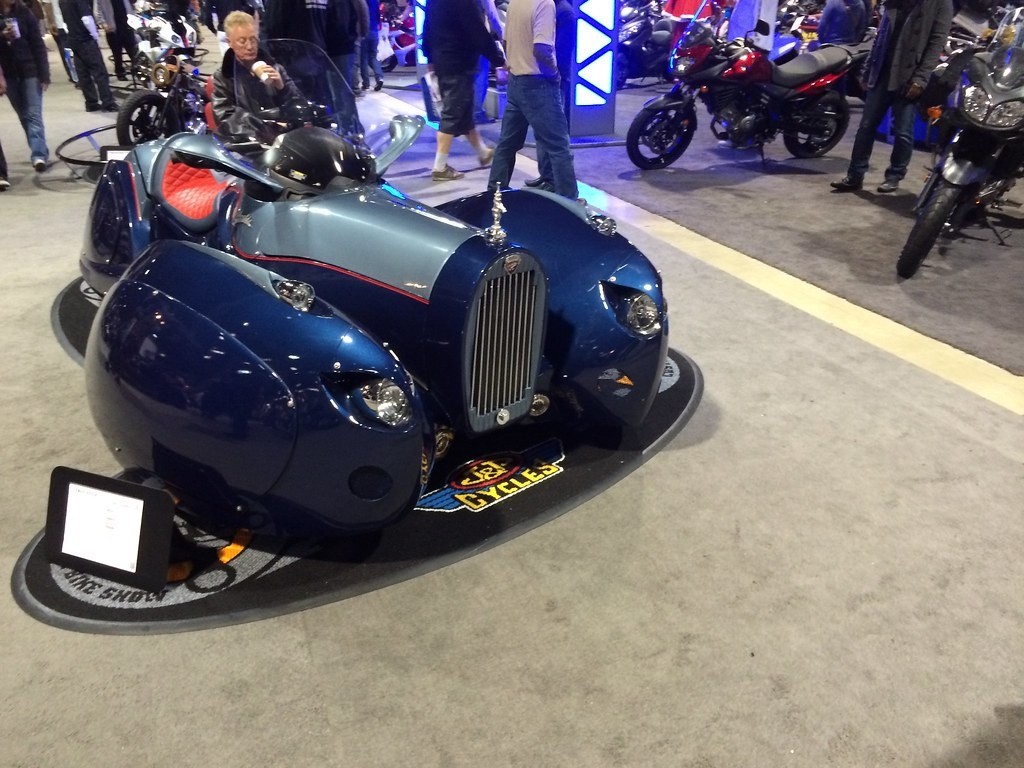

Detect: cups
[3, 18, 21, 39]
[252, 61, 274, 85]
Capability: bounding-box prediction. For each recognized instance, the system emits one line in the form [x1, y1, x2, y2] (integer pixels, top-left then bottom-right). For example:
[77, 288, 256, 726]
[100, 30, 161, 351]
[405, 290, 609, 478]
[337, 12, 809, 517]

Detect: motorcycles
[895, 6, 1024, 281]
[614, 0, 986, 109]
[626, 1, 871, 173]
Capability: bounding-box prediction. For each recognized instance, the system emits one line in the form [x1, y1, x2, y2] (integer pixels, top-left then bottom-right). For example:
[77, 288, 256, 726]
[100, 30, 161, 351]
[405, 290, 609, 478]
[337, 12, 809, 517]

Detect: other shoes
[103, 102, 121, 113]
[361, 84, 371, 92]
[33, 158, 46, 172]
[0, 175, 10, 189]
[86, 103, 102, 112]
[375, 79, 383, 91]
[118, 74, 127, 81]
[353, 89, 365, 97]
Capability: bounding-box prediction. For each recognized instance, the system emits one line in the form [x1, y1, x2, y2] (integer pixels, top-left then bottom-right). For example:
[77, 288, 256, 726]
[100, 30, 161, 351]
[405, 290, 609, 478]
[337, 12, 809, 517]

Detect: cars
[77, 37, 671, 535]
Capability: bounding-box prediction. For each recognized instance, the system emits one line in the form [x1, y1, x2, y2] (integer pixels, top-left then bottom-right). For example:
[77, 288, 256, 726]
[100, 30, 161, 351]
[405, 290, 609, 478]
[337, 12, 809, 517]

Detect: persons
[817, 0, 868, 93]
[0, 0, 587, 207]
[718, 1, 778, 150]
[830, 0, 952, 193]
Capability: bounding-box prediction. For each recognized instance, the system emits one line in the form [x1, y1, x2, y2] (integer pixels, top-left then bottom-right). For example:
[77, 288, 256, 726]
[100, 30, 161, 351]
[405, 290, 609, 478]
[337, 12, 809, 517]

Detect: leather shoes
[538, 182, 556, 194]
[524, 176, 546, 186]
[578, 198, 587, 206]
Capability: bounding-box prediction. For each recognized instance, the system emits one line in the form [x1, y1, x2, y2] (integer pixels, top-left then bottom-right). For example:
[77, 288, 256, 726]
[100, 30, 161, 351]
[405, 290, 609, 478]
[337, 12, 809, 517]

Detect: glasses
[230, 38, 261, 48]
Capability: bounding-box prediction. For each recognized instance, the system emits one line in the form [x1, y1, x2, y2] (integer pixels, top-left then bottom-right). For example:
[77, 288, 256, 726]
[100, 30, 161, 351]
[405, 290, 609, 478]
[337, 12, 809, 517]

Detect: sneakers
[481, 148, 496, 166]
[431, 164, 465, 180]
[831, 177, 863, 191]
[878, 180, 900, 192]
